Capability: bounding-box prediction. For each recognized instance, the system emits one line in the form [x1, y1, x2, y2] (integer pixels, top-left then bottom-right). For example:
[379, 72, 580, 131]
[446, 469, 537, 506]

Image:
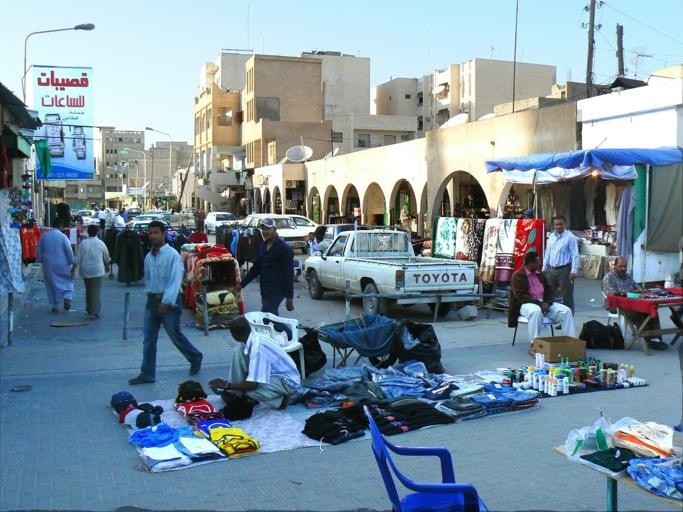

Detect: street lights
[21, 19, 95, 104]
[106, 166, 123, 184]
[144, 125, 172, 210]
[123, 146, 147, 209]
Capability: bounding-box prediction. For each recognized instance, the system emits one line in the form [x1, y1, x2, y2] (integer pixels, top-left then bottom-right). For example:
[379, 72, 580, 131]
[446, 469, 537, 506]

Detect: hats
[258, 217, 276, 229]
[118, 403, 163, 427]
[112, 390, 137, 410]
[175, 379, 207, 403]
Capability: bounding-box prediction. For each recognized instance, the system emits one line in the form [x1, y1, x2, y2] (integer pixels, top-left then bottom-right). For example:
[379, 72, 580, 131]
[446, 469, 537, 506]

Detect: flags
[34, 139, 54, 178]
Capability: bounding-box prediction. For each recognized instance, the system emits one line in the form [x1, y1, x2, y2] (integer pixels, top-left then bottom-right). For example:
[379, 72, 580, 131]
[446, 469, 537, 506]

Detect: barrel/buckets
[294, 257, 303, 282]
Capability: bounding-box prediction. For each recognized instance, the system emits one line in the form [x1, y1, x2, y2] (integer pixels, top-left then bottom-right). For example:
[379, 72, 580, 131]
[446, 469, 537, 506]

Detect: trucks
[301, 228, 480, 318]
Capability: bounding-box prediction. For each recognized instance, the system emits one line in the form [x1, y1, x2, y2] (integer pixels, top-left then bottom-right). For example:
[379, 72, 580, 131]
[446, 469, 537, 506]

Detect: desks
[608, 287, 683, 355]
[553, 445, 683, 512]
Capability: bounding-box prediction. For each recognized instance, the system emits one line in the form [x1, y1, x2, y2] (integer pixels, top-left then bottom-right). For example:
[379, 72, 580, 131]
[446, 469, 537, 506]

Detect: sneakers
[189, 353, 203, 375]
[128, 374, 155, 385]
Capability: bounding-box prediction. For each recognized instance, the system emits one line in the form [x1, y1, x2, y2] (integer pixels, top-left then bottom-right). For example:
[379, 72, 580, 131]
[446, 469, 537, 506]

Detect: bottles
[509, 352, 636, 397]
[671, 420, 683, 452]
[504, 195, 523, 219]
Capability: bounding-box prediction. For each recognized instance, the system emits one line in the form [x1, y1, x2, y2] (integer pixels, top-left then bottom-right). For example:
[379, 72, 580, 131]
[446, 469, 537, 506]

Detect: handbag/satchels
[580, 320, 625, 351]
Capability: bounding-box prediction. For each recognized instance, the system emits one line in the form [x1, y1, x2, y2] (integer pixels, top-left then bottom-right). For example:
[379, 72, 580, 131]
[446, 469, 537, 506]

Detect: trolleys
[293, 312, 399, 369]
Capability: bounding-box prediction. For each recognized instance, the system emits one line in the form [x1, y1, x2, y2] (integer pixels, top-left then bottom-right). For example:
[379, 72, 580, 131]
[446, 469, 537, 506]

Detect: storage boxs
[532, 335, 587, 362]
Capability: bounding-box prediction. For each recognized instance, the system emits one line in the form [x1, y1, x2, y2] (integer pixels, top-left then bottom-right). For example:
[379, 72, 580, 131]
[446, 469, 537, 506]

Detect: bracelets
[226, 382, 232, 390]
[71, 269, 75, 272]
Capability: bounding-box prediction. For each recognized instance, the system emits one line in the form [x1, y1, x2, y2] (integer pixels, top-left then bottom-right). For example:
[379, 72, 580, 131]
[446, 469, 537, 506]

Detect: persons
[208, 316, 301, 409]
[540, 214, 580, 329]
[230, 218, 295, 315]
[72, 224, 112, 318]
[508, 251, 577, 358]
[36, 216, 74, 314]
[170, 207, 206, 233]
[602, 255, 669, 350]
[127, 220, 204, 386]
[98, 207, 128, 229]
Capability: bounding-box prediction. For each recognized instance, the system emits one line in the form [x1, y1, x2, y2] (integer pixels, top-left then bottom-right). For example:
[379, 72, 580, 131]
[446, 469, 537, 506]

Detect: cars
[70, 206, 324, 250]
[306, 223, 370, 258]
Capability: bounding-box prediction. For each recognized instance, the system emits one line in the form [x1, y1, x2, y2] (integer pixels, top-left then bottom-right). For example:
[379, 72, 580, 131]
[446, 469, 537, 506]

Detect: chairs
[511, 313, 561, 347]
[243, 310, 306, 381]
[363, 404, 490, 512]
